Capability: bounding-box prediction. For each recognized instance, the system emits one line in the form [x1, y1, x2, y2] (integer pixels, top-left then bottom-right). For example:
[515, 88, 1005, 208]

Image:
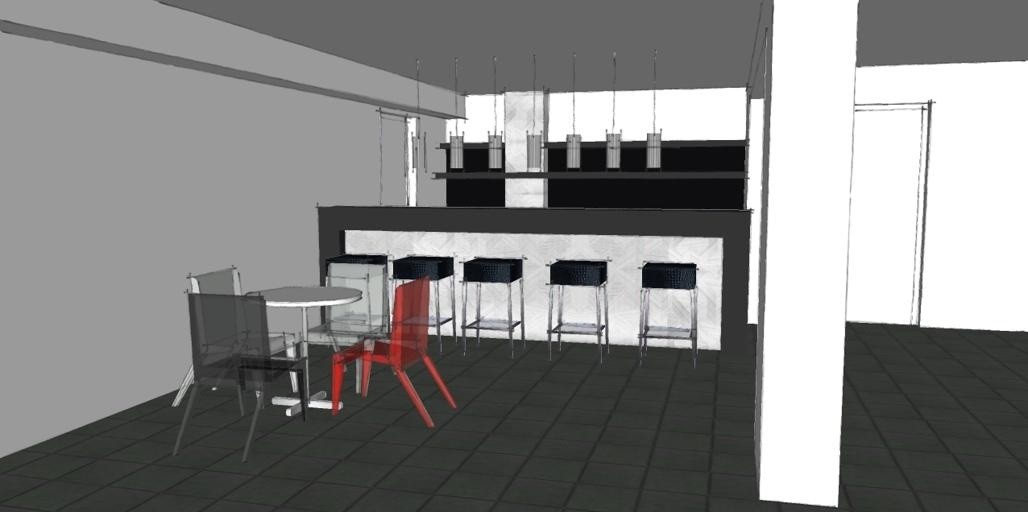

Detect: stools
[324, 253, 391, 350]
[389, 250, 459, 354]
[457, 254, 529, 360]
[544, 255, 612, 366]
[635, 260, 700, 370]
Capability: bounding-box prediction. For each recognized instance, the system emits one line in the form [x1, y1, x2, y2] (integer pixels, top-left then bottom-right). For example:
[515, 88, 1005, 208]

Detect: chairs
[329, 274, 459, 430]
[170, 264, 301, 418]
[170, 287, 309, 465]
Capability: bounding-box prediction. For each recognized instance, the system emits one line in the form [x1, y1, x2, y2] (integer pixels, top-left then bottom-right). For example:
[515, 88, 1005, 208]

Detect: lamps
[449, 57, 464, 170]
[410, 58, 428, 176]
[603, 50, 623, 170]
[525, 53, 543, 170]
[565, 52, 584, 171]
[645, 46, 664, 170]
[487, 55, 503, 170]
